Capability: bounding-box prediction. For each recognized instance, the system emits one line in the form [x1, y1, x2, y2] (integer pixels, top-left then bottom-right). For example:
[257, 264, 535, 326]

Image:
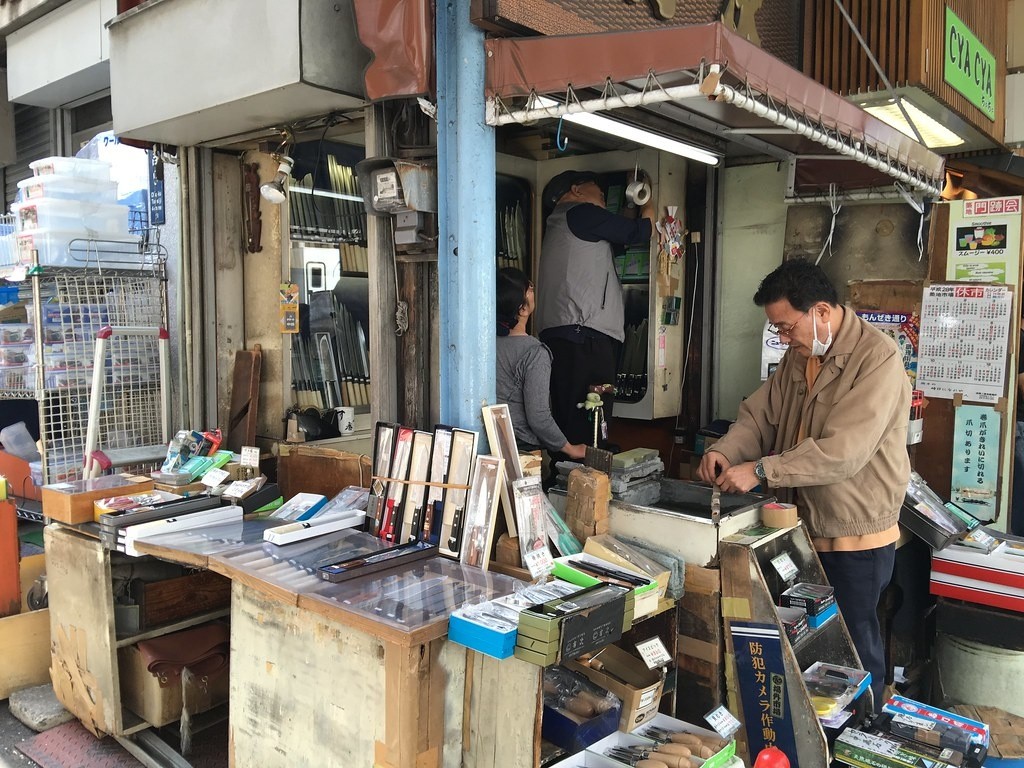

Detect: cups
[334, 407, 355, 436]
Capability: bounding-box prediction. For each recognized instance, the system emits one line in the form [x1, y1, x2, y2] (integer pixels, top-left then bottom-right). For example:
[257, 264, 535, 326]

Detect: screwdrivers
[582, 653, 626, 684]
[580, 647, 607, 668]
[603, 723, 727, 768]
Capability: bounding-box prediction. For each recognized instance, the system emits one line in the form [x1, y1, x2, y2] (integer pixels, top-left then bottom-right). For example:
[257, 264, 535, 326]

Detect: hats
[542, 171, 604, 214]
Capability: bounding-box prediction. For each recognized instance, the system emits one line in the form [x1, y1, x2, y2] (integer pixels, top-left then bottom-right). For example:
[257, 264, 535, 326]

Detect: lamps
[530, 92, 726, 169]
[260, 156, 294, 205]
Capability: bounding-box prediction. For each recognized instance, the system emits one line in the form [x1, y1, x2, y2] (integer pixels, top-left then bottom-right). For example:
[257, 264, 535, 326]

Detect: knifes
[320, 335, 339, 409]
[371, 431, 487, 566]
[290, 333, 324, 408]
[497, 200, 526, 272]
[328, 546, 419, 571]
[330, 575, 504, 624]
[358, 321, 371, 404]
[497, 421, 517, 520]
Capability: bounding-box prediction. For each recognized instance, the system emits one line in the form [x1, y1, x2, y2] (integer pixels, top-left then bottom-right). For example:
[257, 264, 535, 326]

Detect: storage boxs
[0, 156, 153, 390]
[0, 404, 744, 768]
[776, 467, 1024, 768]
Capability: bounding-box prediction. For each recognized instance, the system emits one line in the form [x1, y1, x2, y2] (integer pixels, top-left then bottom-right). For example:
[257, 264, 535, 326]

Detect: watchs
[754, 459, 767, 481]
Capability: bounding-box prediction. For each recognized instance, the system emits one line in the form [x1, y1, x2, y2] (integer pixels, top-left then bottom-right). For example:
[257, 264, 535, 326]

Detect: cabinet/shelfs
[0, 250, 169, 618]
[44, 519, 448, 768]
[719, 517, 874, 768]
[461, 604, 678, 768]
[495, 150, 686, 420]
[243, 117, 372, 439]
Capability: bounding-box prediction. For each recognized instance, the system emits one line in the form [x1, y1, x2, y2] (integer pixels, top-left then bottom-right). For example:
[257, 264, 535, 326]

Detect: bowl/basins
[26, 446, 108, 483]
[0, 304, 162, 389]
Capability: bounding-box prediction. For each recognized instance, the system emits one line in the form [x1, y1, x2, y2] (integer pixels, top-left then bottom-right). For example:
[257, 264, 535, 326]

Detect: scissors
[812, 696, 837, 714]
[805, 681, 847, 698]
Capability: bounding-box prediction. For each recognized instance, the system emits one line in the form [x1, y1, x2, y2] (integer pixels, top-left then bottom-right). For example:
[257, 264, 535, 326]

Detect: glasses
[567, 178, 602, 195]
[525, 280, 534, 299]
[767, 303, 816, 337]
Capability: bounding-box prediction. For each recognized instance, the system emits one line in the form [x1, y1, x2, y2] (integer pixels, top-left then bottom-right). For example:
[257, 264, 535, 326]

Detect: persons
[496, 266, 588, 472]
[537, 170, 656, 492]
[696, 259, 912, 750]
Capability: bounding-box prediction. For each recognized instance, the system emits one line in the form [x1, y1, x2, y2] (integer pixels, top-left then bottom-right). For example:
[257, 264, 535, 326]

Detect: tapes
[625, 181, 651, 205]
[762, 503, 798, 528]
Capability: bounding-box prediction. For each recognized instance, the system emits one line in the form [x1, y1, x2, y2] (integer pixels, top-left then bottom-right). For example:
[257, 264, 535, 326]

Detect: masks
[810, 307, 833, 356]
